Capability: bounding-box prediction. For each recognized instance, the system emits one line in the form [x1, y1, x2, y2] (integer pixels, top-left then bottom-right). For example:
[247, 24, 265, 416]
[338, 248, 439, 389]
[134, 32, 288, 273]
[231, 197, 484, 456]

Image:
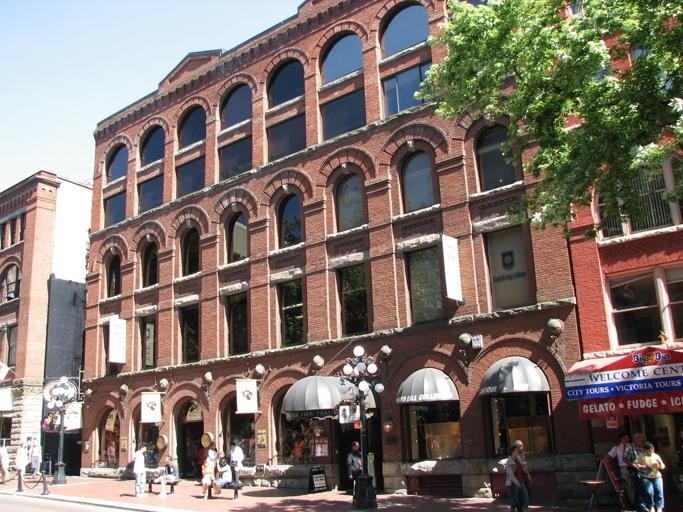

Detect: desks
[578, 479, 607, 512]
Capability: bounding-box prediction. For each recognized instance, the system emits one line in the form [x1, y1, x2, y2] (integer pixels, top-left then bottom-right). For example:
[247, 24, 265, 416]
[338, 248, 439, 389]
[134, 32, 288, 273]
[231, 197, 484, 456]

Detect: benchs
[146, 481, 179, 495]
[195, 482, 243, 500]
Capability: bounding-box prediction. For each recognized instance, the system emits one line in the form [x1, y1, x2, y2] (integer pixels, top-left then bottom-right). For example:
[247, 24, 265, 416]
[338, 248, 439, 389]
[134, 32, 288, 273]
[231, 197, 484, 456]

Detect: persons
[623, 433, 647, 505]
[506, 439, 531, 511]
[134, 444, 146, 498]
[201, 441, 244, 498]
[606, 433, 631, 501]
[107, 439, 116, 466]
[637, 442, 665, 512]
[347, 442, 363, 504]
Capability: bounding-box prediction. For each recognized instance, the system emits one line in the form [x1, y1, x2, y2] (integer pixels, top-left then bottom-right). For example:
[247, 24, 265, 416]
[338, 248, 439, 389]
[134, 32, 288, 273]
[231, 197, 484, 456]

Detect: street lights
[46, 376, 76, 484]
[338, 345, 385, 509]
